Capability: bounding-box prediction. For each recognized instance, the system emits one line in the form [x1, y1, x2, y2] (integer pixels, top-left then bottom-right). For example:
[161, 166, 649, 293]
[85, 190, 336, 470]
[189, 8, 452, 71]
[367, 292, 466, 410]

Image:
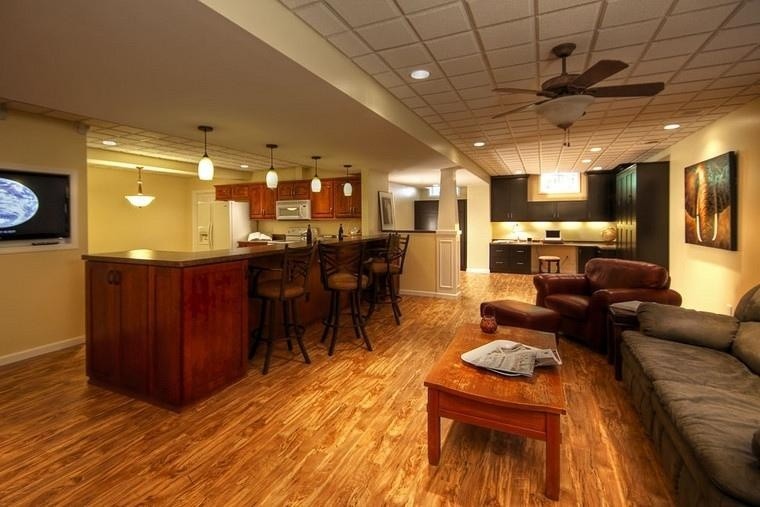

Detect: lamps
[343, 164, 353, 197]
[197, 125, 215, 180]
[265, 144, 279, 189]
[310, 156, 322, 193]
[124, 167, 156, 209]
[535, 94, 595, 147]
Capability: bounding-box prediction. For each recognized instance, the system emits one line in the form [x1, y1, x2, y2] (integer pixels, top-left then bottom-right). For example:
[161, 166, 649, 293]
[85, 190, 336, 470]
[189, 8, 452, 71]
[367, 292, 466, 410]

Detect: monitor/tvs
[0, 169, 70, 241]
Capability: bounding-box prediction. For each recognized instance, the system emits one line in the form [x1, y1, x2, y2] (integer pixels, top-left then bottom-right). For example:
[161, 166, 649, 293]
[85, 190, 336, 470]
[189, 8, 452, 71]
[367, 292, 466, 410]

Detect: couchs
[533, 257, 682, 355]
[619, 283, 760, 507]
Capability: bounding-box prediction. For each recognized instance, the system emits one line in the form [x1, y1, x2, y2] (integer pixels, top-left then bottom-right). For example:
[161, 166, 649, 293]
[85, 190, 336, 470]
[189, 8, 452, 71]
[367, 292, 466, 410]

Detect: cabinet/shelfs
[147, 258, 248, 413]
[309, 178, 334, 220]
[577, 245, 616, 274]
[585, 170, 616, 222]
[490, 242, 531, 275]
[81, 255, 149, 402]
[490, 173, 540, 222]
[615, 161, 669, 272]
[276, 179, 310, 200]
[213, 183, 249, 201]
[248, 181, 278, 220]
[527, 199, 588, 222]
[333, 175, 361, 218]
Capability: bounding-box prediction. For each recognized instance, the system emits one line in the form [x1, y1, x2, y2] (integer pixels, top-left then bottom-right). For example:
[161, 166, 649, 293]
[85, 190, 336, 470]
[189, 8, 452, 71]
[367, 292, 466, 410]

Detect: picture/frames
[377, 190, 397, 232]
[684, 151, 737, 251]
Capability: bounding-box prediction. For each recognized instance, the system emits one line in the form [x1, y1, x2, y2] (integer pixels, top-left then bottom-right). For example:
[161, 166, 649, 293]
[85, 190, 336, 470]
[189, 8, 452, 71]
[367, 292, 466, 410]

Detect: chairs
[247, 238, 318, 375]
[315, 239, 373, 356]
[365, 232, 410, 326]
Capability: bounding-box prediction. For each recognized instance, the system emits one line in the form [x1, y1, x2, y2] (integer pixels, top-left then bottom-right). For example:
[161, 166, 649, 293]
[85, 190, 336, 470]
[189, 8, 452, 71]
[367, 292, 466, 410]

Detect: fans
[490, 42, 664, 119]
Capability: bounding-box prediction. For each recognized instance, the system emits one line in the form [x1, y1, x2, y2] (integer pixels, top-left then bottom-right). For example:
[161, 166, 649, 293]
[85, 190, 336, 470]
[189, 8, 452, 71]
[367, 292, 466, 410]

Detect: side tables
[607, 304, 640, 380]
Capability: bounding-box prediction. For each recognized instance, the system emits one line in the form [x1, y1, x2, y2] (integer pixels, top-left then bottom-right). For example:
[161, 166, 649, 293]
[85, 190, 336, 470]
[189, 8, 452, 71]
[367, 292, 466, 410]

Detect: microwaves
[277, 200, 311, 221]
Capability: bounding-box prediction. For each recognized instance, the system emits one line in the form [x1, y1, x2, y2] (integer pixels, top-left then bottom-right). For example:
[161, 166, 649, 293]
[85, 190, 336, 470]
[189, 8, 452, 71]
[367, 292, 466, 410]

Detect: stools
[538, 255, 560, 273]
[479, 300, 561, 347]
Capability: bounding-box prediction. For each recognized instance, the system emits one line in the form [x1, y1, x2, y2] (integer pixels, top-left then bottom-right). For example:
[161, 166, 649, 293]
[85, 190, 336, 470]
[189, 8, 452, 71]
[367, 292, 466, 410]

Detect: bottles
[338, 225, 343, 240]
[307, 225, 312, 245]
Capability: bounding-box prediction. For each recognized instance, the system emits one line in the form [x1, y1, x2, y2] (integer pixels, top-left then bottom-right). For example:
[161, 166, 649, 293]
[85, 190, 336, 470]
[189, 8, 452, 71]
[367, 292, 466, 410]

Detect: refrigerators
[193, 201, 258, 252]
